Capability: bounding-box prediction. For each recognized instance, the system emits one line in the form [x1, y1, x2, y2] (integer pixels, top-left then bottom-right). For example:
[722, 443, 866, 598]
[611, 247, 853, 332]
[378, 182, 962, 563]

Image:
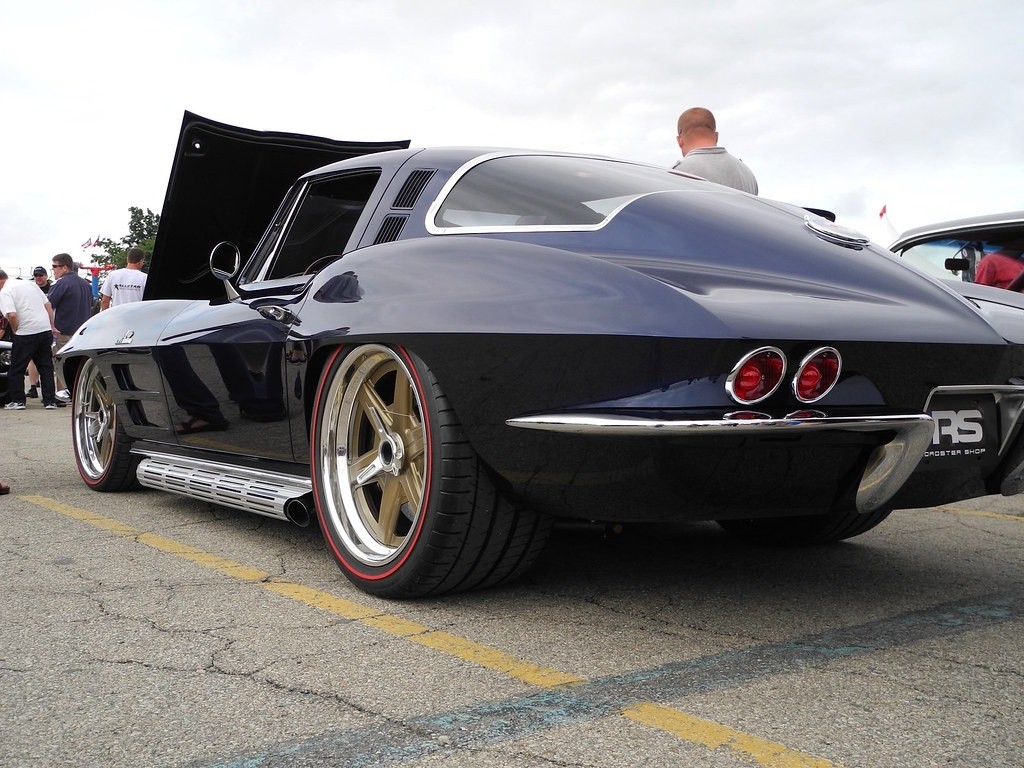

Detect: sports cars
[54, 111, 1024, 598]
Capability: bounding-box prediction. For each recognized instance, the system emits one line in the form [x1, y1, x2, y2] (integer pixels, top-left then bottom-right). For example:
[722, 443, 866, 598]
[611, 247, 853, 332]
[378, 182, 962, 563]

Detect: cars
[887, 210, 1024, 387]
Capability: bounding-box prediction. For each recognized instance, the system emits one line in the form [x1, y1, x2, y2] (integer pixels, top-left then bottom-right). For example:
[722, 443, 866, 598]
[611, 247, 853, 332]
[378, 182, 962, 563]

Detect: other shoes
[0, 481, 10, 493]
[25, 385, 38, 398]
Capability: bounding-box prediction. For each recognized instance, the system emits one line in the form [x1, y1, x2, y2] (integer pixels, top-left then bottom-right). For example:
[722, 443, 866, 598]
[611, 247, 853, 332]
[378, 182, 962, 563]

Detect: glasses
[52, 265, 68, 268]
[35, 274, 43, 277]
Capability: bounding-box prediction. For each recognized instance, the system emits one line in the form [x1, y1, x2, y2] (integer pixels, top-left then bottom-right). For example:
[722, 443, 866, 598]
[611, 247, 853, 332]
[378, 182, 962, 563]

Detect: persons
[0, 253, 101, 410]
[672, 107, 759, 198]
[99, 247, 148, 313]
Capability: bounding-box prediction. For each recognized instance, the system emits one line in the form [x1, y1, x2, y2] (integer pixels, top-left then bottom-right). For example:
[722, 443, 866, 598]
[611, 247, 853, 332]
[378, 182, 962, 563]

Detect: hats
[33, 267, 47, 275]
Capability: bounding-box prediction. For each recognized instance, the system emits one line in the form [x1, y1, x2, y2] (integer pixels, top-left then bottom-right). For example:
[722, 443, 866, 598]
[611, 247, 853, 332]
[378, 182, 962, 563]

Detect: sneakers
[4, 402, 26, 410]
[46, 403, 58, 408]
